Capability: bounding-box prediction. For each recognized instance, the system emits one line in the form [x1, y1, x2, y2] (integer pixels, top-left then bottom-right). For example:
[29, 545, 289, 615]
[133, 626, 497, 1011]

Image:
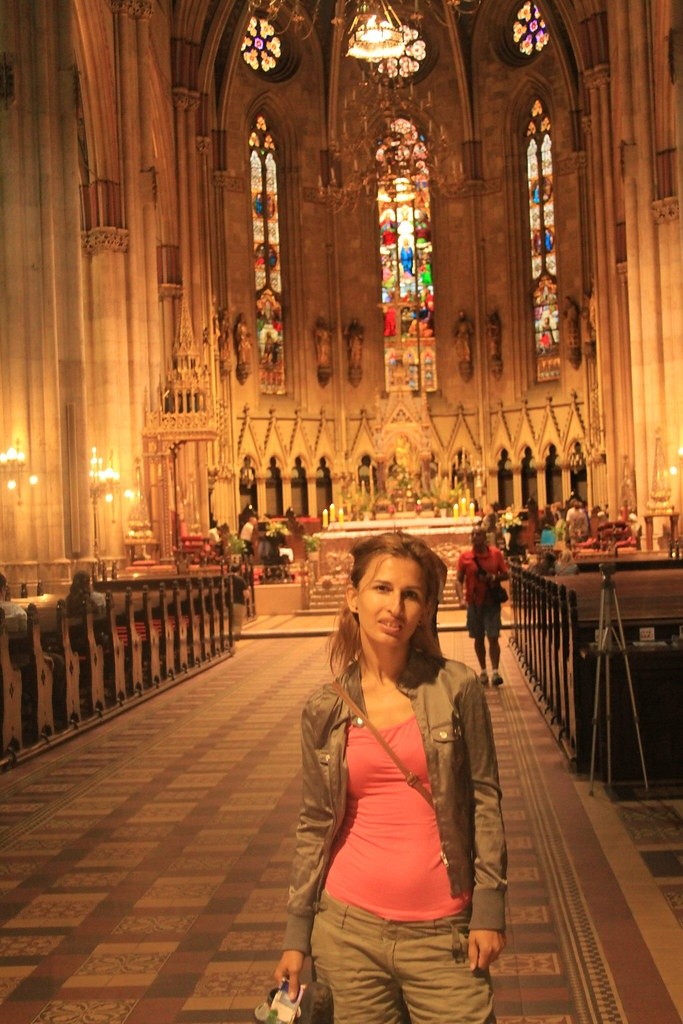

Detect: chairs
[169, 511, 212, 565]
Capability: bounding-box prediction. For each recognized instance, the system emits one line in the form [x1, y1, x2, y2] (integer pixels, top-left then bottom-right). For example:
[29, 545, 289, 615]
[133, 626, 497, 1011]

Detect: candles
[461, 497, 467, 515]
[323, 508, 328, 526]
[338, 508, 344, 524]
[453, 504, 458, 520]
[329, 503, 336, 520]
[469, 503, 475, 518]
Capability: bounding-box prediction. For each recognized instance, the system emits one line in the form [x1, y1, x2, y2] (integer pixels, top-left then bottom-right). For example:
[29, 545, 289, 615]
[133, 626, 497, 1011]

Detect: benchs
[0, 575, 236, 770]
[509, 555, 683, 776]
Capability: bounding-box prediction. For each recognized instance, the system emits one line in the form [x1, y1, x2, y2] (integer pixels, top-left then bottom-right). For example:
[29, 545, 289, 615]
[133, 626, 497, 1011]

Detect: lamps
[248, 0, 483, 212]
[0, 448, 39, 506]
[90, 446, 131, 557]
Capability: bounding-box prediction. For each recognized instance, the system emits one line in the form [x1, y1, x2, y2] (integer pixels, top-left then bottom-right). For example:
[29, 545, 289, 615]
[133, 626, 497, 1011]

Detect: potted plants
[224, 533, 248, 566]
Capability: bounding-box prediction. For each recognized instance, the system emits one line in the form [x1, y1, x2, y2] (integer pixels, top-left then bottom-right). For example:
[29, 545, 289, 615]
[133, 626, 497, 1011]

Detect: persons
[481, 492, 642, 609]
[454, 527, 509, 687]
[273, 531, 507, 1024]
[0, 572, 117, 708]
[186, 514, 257, 566]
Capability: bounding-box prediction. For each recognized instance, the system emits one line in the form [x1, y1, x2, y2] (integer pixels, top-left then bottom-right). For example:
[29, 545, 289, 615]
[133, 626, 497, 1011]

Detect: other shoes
[480, 673, 488, 684]
[491, 672, 503, 686]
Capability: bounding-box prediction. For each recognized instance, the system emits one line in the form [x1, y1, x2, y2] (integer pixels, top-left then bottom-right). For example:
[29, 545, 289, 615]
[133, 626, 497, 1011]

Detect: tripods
[589, 561, 652, 797]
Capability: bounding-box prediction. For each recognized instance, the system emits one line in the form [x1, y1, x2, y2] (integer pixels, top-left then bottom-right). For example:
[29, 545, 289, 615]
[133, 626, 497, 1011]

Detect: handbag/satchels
[489, 580, 508, 603]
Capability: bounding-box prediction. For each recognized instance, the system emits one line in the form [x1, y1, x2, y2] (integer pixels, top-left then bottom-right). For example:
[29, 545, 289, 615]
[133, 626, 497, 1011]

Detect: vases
[307, 551, 318, 560]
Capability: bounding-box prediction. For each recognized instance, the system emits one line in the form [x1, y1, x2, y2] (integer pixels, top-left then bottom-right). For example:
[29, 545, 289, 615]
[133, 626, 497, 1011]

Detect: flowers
[265, 520, 292, 538]
[497, 508, 522, 529]
[301, 534, 325, 550]
